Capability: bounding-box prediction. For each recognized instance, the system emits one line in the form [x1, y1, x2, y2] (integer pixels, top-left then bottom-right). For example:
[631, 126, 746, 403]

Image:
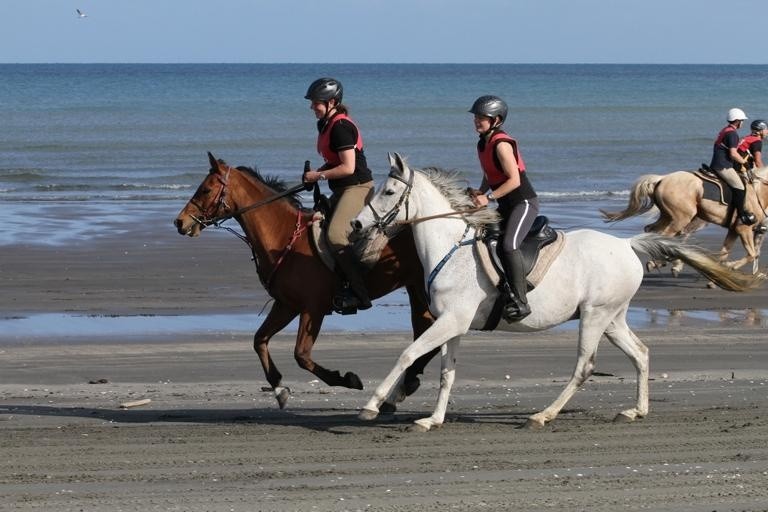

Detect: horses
[350, 151, 768, 433]
[173, 151, 442, 415]
[599, 165, 768, 290]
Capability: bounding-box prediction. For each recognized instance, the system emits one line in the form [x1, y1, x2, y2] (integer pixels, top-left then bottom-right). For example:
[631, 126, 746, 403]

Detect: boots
[336, 249, 372, 315]
[502, 247, 534, 324]
[735, 188, 757, 224]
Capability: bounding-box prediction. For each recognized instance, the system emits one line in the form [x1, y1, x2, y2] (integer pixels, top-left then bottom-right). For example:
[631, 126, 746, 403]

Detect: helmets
[304, 77, 343, 106]
[750, 119, 768, 131]
[725, 107, 750, 122]
[467, 93, 510, 126]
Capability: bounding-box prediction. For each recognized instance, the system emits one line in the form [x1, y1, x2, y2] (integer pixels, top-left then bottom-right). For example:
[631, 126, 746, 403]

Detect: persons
[466, 94, 540, 320]
[731, 119, 767, 175]
[300, 77, 376, 312]
[707, 108, 767, 235]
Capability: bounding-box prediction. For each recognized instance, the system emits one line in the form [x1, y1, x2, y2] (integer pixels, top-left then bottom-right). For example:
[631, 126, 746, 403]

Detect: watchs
[318, 172, 326, 181]
[487, 193, 496, 201]
